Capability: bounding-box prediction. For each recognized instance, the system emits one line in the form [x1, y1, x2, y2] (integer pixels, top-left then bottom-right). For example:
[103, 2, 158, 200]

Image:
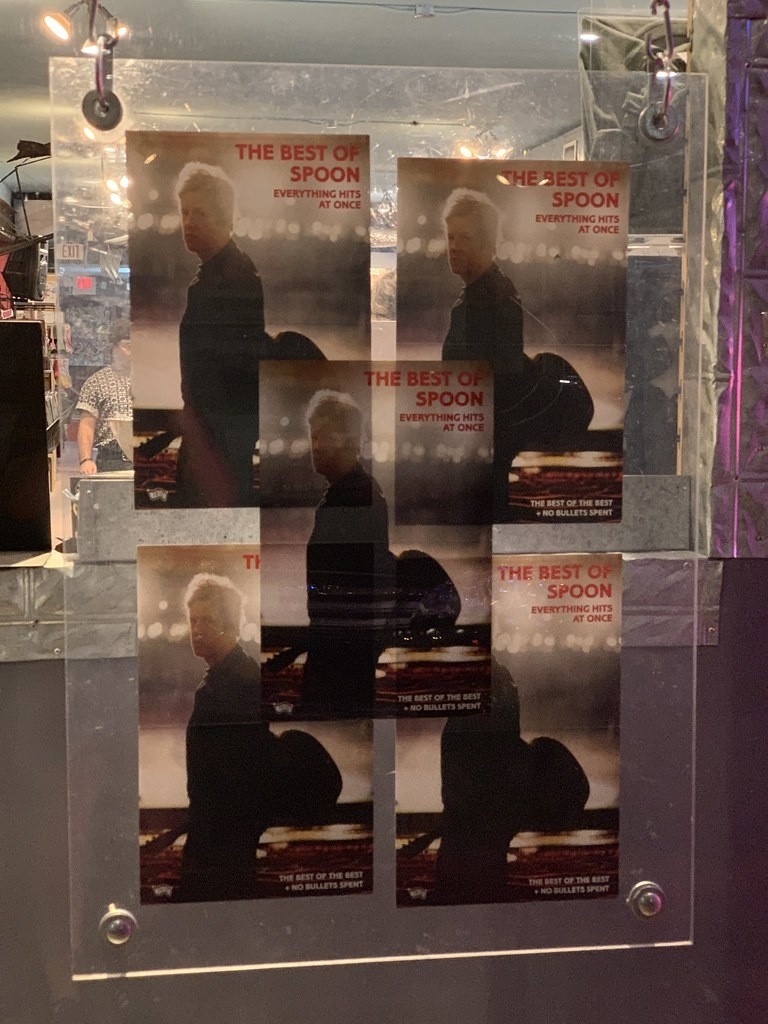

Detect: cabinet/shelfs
[0, 298, 56, 492]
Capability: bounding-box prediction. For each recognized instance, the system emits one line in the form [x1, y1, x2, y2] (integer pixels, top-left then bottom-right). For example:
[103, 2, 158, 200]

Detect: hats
[109, 319, 129, 342]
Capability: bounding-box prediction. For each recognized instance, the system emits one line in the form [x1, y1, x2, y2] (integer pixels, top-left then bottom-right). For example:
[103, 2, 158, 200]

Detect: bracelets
[80, 458, 93, 464]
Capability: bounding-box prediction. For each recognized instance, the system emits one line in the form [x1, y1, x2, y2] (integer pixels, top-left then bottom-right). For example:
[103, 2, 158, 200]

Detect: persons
[184, 573, 266, 901]
[75, 318, 132, 475]
[294, 391, 389, 719]
[432, 656, 520, 902]
[443, 189, 525, 463]
[179, 163, 265, 505]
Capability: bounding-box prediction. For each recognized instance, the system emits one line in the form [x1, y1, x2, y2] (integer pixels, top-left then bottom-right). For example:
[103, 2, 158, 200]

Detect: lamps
[42, 0, 130, 55]
